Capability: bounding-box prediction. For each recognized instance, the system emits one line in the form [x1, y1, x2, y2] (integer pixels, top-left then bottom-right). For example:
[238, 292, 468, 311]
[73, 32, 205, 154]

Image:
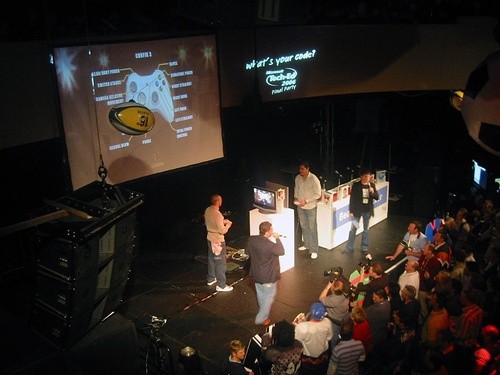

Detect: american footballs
[109, 102, 156, 136]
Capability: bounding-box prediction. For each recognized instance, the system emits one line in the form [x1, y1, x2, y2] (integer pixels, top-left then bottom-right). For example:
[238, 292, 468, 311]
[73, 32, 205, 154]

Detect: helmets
[310, 301, 326, 320]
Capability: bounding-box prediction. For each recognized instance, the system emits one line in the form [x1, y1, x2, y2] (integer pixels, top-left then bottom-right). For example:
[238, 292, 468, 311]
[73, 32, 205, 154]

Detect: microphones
[368, 183, 372, 193]
[280, 235, 287, 238]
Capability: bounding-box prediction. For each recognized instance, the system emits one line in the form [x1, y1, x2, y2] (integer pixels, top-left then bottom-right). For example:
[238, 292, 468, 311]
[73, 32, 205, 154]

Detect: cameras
[358, 261, 370, 273]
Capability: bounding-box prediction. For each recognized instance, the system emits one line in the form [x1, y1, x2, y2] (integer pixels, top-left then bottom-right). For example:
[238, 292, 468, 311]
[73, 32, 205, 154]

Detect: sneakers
[311, 251, 318, 259]
[361, 248, 369, 255]
[341, 248, 353, 254]
[264, 319, 271, 325]
[298, 245, 306, 250]
[215, 284, 233, 291]
[207, 278, 217, 285]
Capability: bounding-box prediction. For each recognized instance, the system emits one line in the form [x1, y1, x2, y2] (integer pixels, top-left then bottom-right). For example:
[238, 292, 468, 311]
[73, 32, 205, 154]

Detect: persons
[293, 163, 321, 259]
[245, 222, 284, 325]
[205, 194, 233, 291]
[229, 181, 500, 375]
[341, 171, 379, 254]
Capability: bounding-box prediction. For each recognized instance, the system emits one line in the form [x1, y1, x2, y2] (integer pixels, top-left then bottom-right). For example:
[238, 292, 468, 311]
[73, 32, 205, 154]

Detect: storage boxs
[317, 170, 386, 207]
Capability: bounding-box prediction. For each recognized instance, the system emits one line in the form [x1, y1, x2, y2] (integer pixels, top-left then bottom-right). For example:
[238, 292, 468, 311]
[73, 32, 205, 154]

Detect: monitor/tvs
[252, 186, 278, 214]
[265, 181, 289, 208]
